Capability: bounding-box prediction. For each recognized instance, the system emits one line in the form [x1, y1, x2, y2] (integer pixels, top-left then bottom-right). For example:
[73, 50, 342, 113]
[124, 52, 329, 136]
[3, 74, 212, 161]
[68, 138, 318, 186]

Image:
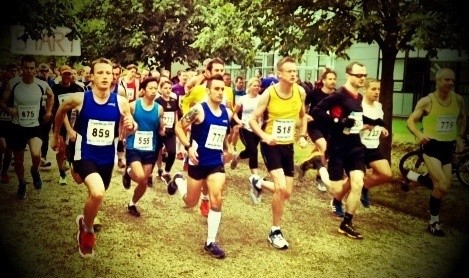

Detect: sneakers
[338, 221, 362, 239]
[30, 166, 42, 189]
[93, 216, 102, 232]
[360, 186, 370, 207]
[59, 174, 67, 185]
[400, 168, 414, 192]
[128, 203, 140, 217]
[329, 198, 344, 217]
[294, 162, 305, 182]
[122, 166, 132, 189]
[267, 228, 289, 249]
[17, 180, 27, 199]
[76, 215, 95, 259]
[428, 220, 445, 237]
[38, 158, 51, 171]
[166, 172, 183, 195]
[315, 175, 327, 192]
[204, 241, 225, 258]
[200, 199, 210, 216]
[249, 174, 263, 204]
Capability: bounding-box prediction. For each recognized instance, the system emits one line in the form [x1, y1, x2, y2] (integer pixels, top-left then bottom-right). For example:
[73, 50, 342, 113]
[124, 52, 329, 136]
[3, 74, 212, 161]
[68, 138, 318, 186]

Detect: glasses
[39, 69, 49, 73]
[347, 73, 368, 78]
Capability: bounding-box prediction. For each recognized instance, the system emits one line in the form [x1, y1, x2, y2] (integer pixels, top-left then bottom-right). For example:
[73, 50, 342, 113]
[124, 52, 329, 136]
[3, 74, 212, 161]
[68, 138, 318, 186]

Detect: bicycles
[399, 116, 469, 188]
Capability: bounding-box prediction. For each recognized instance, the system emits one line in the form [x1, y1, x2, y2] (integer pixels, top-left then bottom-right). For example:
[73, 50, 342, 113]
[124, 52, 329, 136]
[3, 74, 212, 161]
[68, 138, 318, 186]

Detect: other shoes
[161, 151, 188, 162]
[158, 169, 164, 180]
[118, 161, 125, 168]
[230, 156, 238, 170]
[0, 147, 29, 172]
[147, 176, 152, 187]
[161, 174, 172, 186]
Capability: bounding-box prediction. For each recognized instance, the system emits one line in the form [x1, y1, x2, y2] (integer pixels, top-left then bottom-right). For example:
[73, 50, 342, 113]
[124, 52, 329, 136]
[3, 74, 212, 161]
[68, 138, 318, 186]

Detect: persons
[51, 58, 138, 258]
[0, 54, 314, 215]
[402, 68, 469, 237]
[248, 57, 307, 250]
[305, 60, 394, 239]
[167, 76, 233, 262]
